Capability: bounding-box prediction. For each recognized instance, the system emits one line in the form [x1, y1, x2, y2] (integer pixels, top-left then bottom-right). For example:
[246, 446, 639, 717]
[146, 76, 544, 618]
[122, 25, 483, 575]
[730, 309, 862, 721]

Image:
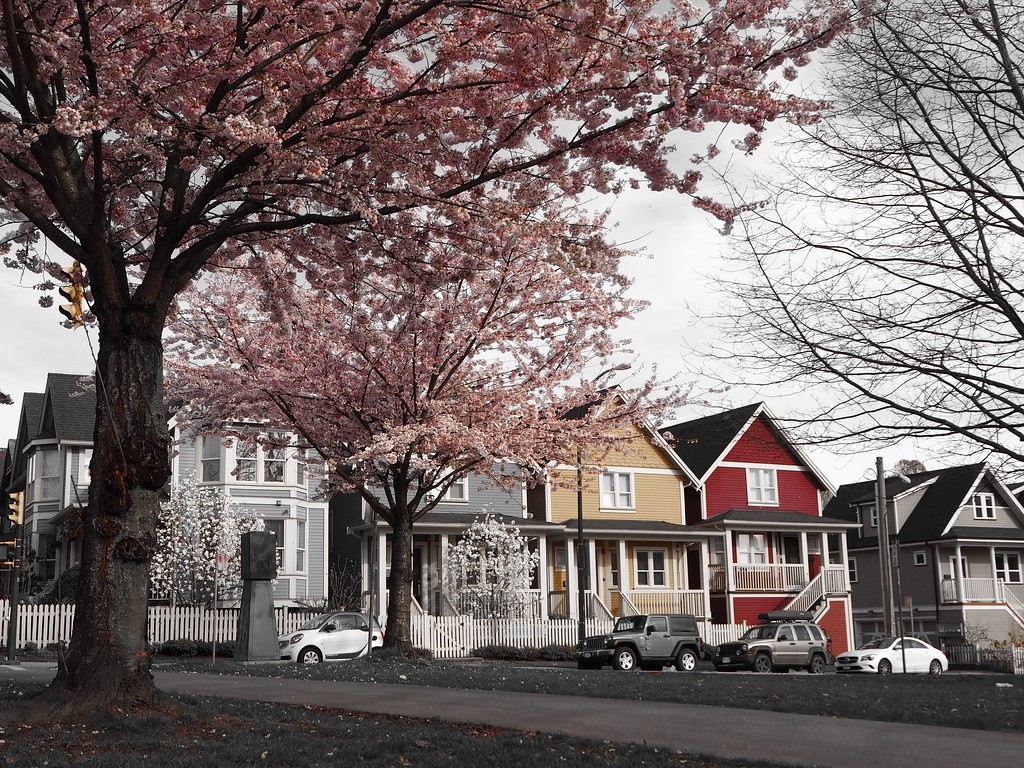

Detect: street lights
[576, 361, 631, 645]
[875, 456, 911, 636]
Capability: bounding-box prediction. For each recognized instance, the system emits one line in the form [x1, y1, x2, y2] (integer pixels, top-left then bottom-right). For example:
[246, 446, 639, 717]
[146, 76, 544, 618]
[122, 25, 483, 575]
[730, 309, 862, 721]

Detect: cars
[277, 611, 387, 664]
[836, 636, 950, 675]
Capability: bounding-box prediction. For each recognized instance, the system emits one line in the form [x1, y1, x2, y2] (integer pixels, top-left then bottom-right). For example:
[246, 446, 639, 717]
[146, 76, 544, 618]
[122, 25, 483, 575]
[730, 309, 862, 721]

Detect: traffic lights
[56, 262, 82, 331]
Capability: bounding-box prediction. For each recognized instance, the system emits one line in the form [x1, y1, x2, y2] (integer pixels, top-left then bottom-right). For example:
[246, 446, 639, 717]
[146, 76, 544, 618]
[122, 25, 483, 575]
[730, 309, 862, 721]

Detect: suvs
[713, 620, 835, 672]
[573, 612, 702, 672]
